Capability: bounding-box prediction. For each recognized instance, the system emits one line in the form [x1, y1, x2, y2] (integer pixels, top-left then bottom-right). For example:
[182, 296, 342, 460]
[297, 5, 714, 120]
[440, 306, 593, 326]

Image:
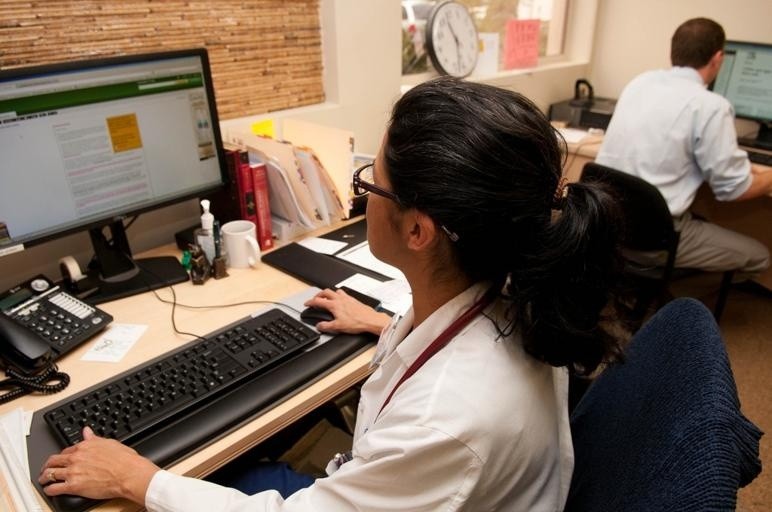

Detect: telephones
[0, 273, 113, 376]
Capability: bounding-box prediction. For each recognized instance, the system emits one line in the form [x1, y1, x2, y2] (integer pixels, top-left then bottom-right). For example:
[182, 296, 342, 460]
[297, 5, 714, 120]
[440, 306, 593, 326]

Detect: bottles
[194, 198, 227, 266]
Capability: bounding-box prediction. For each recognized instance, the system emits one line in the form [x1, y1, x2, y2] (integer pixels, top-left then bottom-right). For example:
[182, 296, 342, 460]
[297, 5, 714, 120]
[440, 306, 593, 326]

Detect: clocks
[424, 0, 481, 79]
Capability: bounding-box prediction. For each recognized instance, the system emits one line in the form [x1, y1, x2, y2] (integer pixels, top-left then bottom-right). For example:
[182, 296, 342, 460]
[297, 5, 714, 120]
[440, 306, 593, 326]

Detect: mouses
[300, 306, 334, 327]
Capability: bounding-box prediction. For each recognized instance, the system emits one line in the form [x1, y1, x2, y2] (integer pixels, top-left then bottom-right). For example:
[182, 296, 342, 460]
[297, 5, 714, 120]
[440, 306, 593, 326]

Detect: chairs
[577, 160, 734, 335]
[563, 296, 764, 511]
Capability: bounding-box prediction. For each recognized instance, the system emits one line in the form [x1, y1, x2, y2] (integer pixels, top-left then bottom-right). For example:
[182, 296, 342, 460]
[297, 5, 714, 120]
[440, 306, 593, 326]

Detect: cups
[221, 220, 261, 269]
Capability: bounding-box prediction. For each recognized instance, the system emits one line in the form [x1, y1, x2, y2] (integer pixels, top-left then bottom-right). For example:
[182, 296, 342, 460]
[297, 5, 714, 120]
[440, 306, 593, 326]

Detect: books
[222, 119, 412, 252]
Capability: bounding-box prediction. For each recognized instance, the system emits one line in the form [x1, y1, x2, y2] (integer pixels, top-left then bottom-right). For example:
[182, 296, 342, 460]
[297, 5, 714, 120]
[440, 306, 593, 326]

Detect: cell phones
[340, 286, 381, 311]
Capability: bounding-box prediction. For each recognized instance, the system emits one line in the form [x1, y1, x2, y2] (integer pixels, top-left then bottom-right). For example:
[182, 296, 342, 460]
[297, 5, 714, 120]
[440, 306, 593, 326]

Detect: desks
[0, 217, 413, 510]
[550, 120, 772, 292]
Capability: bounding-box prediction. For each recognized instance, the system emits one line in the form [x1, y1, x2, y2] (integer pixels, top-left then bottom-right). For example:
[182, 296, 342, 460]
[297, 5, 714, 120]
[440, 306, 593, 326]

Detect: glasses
[353, 163, 459, 242]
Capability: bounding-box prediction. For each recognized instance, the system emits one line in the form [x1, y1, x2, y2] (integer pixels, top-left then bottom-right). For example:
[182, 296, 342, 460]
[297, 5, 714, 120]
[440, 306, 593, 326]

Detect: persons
[29, 72, 660, 511]
[592, 16, 771, 304]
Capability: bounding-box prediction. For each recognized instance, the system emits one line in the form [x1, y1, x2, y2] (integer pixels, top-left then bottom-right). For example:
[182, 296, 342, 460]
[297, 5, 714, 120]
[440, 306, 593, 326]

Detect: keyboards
[44, 308, 321, 451]
[745, 150, 772, 167]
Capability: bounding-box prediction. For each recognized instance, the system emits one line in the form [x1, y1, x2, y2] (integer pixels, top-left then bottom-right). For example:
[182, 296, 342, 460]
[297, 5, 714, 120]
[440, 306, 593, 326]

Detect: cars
[401, 0, 437, 62]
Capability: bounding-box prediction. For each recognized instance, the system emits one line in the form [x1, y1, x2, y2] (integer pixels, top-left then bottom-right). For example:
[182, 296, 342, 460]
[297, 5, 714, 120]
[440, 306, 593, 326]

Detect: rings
[48, 468, 57, 481]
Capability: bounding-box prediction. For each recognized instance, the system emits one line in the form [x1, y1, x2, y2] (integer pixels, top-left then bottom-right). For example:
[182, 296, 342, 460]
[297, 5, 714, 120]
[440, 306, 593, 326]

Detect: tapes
[58, 256, 81, 282]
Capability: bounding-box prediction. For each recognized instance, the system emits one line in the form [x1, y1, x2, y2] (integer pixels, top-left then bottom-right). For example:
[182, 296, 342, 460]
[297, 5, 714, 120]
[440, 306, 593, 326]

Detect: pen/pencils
[213, 221, 221, 258]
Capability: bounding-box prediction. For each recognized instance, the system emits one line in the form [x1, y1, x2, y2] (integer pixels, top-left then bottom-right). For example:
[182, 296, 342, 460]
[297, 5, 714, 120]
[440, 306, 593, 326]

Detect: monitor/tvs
[0, 46, 232, 306]
[707, 40, 772, 150]
